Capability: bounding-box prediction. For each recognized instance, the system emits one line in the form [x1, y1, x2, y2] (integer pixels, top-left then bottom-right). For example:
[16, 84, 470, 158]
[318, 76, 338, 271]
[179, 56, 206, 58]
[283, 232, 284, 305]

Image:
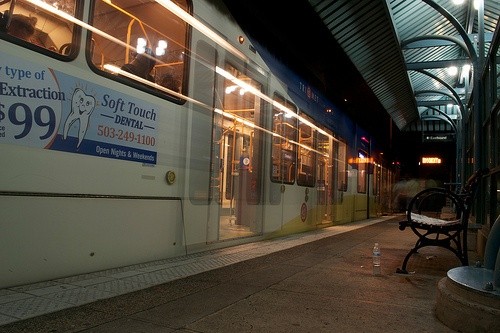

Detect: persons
[0, 10, 38, 42]
[161, 70, 181, 94]
[118, 47, 156, 86]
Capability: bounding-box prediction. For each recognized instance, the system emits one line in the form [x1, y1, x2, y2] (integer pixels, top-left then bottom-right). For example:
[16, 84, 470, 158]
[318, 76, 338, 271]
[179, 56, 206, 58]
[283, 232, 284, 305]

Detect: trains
[0, 0, 396, 289]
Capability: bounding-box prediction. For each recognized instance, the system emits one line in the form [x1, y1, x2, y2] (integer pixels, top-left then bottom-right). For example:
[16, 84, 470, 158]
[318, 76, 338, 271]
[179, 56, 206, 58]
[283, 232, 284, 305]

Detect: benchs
[394, 166, 486, 271]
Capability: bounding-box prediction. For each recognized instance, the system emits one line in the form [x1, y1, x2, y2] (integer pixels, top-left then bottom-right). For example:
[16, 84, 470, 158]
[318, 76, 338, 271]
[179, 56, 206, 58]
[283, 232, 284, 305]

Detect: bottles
[372, 243, 381, 267]
